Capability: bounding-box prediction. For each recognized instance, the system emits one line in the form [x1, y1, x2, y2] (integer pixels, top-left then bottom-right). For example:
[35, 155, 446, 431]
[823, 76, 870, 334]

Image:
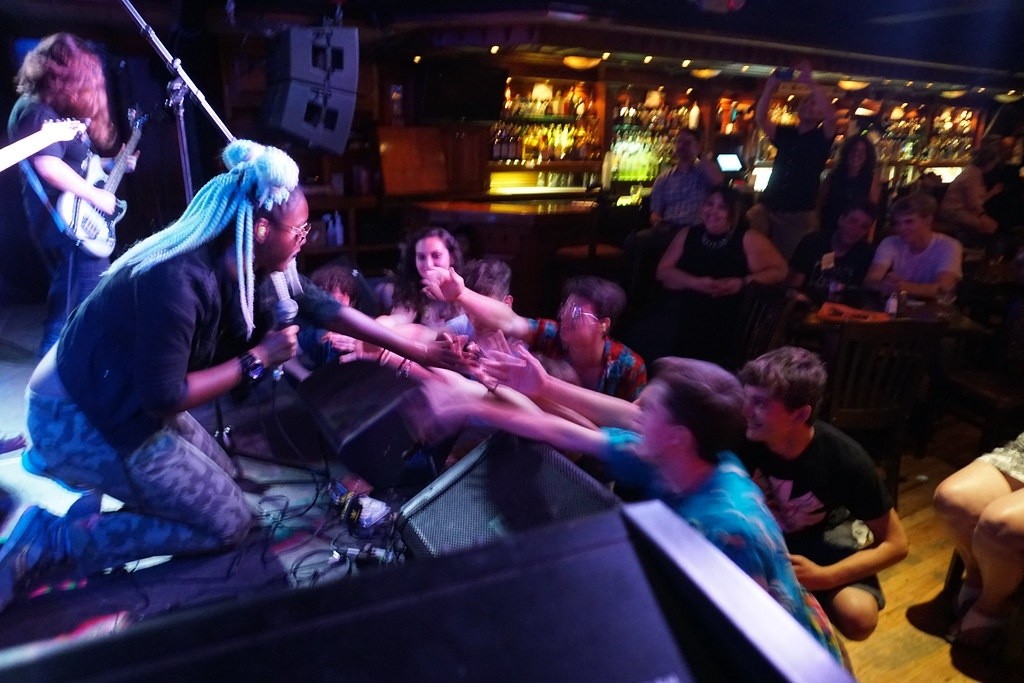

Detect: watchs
[235, 351, 264, 388]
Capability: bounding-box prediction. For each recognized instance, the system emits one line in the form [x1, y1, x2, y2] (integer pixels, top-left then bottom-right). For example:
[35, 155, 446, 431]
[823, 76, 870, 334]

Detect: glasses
[260, 217, 312, 245]
[555, 302, 600, 324]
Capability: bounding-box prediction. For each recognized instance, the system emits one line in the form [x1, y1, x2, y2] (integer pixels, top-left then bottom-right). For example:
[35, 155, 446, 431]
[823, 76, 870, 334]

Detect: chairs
[713, 279, 1024, 511]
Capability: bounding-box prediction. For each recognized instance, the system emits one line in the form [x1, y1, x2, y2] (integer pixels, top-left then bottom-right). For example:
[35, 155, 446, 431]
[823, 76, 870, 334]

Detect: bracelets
[488, 382, 499, 393]
[380, 349, 390, 368]
[395, 358, 412, 382]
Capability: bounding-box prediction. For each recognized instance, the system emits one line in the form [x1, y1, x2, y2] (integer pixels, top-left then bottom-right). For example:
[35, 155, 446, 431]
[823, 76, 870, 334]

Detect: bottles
[493, 82, 974, 187]
[327, 207, 343, 246]
[885, 292, 898, 314]
[898, 291, 907, 314]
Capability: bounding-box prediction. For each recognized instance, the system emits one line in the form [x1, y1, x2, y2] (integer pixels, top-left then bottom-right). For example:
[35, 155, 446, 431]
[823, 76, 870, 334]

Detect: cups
[630, 185, 642, 205]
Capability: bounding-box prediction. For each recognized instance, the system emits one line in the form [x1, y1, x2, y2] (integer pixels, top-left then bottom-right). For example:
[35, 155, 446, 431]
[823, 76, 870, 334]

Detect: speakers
[0, 499, 855, 683]
[256, 24, 360, 154]
[297, 350, 620, 558]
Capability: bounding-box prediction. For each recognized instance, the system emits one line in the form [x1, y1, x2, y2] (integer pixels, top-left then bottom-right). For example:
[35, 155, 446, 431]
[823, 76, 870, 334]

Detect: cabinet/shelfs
[485, 113, 974, 170]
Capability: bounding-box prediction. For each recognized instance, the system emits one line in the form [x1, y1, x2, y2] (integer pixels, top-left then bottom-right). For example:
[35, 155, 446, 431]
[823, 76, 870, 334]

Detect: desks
[788, 307, 994, 459]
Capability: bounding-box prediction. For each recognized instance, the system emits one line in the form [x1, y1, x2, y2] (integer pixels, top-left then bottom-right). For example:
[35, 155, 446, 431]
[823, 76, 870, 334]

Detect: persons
[7, 29, 141, 364]
[0, 136, 479, 616]
[297, 61, 1024, 683]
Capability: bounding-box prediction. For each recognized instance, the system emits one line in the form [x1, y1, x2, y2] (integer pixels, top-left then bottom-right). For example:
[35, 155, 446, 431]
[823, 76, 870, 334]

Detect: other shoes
[0, 503, 59, 609]
[906, 593, 971, 644]
[20, 444, 97, 494]
[948, 598, 1012, 665]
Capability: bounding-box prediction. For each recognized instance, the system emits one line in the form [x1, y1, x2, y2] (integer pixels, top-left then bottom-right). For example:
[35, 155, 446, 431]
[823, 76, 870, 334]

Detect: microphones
[268, 299, 299, 392]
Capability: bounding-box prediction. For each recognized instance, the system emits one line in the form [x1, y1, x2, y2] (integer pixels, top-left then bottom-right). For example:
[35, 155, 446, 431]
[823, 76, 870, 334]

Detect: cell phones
[775, 71, 793, 79]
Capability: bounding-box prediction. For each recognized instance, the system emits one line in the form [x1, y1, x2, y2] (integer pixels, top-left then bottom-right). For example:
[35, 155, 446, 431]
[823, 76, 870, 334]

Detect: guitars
[54, 118, 143, 262]
[0, 117, 87, 174]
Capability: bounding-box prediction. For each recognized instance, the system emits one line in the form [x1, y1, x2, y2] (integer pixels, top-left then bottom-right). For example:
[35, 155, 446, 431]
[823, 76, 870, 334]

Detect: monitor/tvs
[419, 60, 506, 123]
[717, 154, 743, 172]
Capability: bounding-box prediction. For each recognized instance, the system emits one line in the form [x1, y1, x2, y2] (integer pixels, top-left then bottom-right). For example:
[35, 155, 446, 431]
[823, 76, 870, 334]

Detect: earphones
[257, 226, 266, 236]
